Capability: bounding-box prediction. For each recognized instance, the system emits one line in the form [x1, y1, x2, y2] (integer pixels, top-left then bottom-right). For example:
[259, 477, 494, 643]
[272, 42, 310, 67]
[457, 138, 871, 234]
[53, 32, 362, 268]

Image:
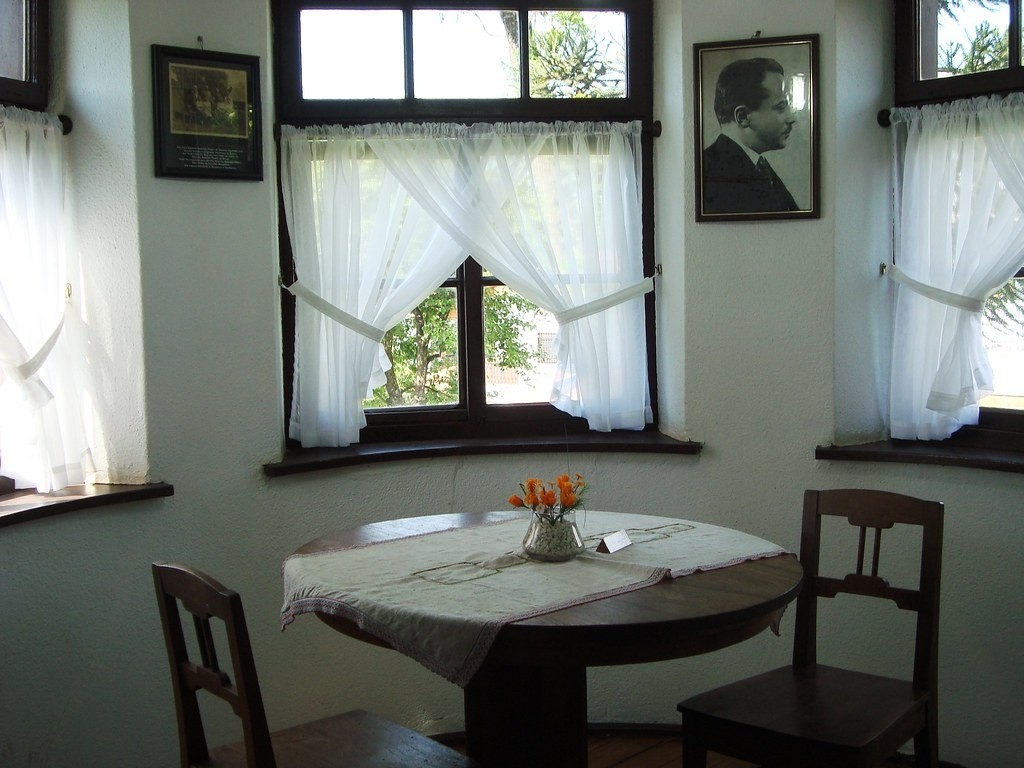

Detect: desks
[273, 511, 804, 768]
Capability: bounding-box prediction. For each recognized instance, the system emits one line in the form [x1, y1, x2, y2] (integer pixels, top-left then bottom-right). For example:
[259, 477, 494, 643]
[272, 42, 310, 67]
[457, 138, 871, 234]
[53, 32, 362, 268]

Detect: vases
[522, 513, 588, 563]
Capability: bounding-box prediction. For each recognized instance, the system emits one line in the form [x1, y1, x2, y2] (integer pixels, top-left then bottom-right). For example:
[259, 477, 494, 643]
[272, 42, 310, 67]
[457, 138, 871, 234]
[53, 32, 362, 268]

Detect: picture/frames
[692, 33, 820, 222]
[150, 41, 266, 181]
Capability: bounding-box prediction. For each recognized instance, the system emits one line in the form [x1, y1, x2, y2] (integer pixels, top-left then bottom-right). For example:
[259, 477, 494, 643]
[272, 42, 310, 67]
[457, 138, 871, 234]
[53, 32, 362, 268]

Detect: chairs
[675, 485, 945, 767]
[150, 560, 473, 768]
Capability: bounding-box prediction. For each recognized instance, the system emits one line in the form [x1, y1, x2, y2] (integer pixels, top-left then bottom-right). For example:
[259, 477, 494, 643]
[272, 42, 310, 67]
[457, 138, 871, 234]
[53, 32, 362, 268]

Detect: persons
[188, 81, 235, 126]
[704, 58, 800, 211]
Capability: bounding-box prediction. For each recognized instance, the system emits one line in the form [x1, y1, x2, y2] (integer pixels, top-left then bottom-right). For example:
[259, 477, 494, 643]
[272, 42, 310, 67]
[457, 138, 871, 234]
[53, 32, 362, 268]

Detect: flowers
[506, 475, 592, 522]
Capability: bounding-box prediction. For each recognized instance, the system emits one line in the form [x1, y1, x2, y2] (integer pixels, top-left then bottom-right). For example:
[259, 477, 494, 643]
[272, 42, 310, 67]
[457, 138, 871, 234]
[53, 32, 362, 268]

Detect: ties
[757, 155, 772, 187]
